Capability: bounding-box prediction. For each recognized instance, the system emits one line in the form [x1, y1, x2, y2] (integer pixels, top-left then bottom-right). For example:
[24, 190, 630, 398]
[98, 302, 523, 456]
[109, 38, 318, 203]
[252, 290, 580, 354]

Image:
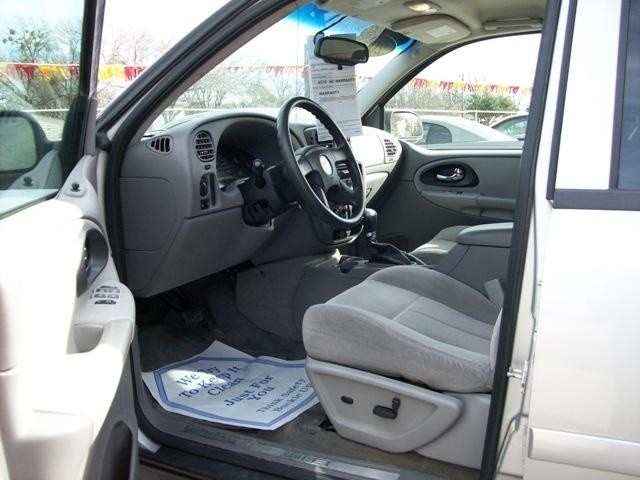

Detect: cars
[387, 109, 530, 149]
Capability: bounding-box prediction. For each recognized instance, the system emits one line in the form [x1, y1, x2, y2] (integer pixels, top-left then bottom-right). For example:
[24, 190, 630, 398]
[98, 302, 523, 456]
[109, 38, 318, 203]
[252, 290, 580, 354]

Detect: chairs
[302, 264, 504, 465]
[409, 227, 515, 298]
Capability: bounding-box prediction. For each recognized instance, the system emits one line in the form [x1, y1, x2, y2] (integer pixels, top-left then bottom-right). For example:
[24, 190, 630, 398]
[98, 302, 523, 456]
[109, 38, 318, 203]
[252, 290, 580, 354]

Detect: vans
[0, 0, 639, 479]
